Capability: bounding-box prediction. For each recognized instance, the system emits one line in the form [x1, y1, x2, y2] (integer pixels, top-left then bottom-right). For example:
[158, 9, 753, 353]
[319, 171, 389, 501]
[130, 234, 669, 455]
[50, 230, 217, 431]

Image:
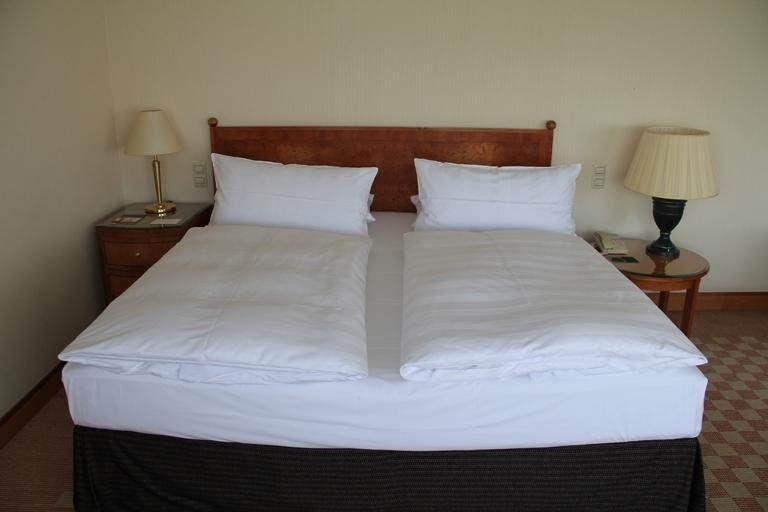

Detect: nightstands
[95, 201, 214, 306]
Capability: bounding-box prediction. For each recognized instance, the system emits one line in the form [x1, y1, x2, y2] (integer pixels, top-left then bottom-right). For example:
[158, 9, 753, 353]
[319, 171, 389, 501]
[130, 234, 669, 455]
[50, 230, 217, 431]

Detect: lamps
[622, 126, 721, 258]
[124, 109, 183, 214]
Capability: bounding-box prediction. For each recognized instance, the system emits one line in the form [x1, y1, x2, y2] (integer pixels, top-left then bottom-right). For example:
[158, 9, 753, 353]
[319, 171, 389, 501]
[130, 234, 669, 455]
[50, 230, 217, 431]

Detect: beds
[56, 118, 708, 512]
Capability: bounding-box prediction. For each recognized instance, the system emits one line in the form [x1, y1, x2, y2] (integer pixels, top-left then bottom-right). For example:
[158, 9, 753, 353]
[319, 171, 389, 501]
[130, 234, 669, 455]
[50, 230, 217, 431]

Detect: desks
[590, 237, 710, 340]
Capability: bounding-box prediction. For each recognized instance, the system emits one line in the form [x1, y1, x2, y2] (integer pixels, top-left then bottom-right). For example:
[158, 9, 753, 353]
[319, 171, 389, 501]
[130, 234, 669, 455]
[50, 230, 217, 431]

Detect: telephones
[592, 230, 628, 255]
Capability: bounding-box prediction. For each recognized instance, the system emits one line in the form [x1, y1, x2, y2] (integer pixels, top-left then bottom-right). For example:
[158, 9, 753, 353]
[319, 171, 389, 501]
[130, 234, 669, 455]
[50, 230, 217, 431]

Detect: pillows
[408, 156, 584, 239]
[207, 154, 383, 242]
